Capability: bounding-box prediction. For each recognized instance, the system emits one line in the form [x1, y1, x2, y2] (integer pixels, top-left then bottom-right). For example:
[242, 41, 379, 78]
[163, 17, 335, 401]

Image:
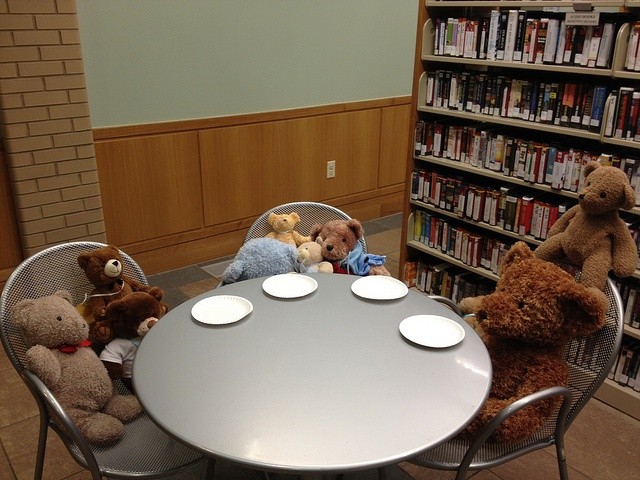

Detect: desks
[131, 272, 494, 474]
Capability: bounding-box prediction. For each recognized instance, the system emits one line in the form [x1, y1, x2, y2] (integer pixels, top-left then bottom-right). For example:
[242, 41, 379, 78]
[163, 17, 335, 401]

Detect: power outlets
[327, 160, 336, 179]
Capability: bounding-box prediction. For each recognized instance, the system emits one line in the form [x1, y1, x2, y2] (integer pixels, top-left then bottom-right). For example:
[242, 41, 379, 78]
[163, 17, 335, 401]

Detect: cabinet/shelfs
[398, 1, 640, 422]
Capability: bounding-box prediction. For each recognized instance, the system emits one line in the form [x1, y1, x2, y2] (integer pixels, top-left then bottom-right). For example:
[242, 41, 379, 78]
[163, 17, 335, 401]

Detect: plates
[398, 314, 466, 349]
[262, 273, 319, 299]
[191, 294, 254, 325]
[350, 274, 409, 300]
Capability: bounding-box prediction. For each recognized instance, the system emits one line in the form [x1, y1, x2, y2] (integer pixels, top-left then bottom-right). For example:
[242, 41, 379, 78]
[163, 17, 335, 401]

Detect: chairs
[0, 242, 210, 480]
[403, 265, 624, 478]
[217, 202, 369, 289]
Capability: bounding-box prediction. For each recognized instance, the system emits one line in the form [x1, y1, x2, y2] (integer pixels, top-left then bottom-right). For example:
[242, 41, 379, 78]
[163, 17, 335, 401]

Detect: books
[404, 252, 496, 303]
[587, 151, 624, 169]
[433, 8, 614, 67]
[606, 278, 639, 329]
[411, 168, 568, 241]
[566, 330, 640, 393]
[414, 210, 513, 276]
[625, 159, 640, 206]
[625, 19, 640, 72]
[416, 121, 586, 194]
[426, 69, 640, 143]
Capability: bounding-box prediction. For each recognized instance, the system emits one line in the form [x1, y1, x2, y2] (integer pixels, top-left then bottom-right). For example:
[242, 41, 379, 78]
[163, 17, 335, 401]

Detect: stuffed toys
[459, 295, 493, 344]
[98, 287, 164, 395]
[76, 244, 169, 343]
[220, 237, 318, 285]
[458, 240, 606, 444]
[310, 218, 391, 276]
[532, 159, 638, 292]
[10, 289, 143, 445]
[297, 236, 334, 274]
[264, 212, 311, 248]
